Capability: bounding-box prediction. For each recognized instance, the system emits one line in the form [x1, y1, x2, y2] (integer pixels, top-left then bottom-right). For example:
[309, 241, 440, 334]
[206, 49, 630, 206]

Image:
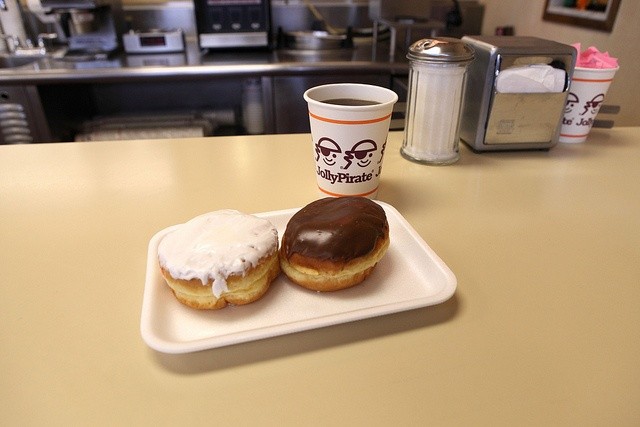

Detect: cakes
[278, 196, 392, 292]
[155, 209, 281, 309]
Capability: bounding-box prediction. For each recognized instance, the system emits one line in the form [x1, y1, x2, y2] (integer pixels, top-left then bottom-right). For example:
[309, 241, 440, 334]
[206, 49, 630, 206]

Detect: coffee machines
[26, 1, 120, 62]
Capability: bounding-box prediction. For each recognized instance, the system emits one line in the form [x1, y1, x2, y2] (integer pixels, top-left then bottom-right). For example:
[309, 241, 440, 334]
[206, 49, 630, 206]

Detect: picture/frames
[541, 1, 621, 33]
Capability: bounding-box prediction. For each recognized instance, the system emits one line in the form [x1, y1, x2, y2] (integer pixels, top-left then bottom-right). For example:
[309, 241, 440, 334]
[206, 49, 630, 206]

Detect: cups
[559, 62, 620, 145]
[302, 82, 399, 199]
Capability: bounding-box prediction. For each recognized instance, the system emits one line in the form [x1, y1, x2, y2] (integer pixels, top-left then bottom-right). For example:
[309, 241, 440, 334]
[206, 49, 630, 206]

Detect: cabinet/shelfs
[273, 69, 412, 135]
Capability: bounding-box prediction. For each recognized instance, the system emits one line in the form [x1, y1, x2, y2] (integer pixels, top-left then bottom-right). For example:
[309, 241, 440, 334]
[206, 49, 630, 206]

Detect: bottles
[400, 37, 478, 167]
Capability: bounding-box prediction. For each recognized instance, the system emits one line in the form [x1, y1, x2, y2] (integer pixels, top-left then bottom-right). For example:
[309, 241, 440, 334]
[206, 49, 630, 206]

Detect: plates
[139, 199, 459, 355]
